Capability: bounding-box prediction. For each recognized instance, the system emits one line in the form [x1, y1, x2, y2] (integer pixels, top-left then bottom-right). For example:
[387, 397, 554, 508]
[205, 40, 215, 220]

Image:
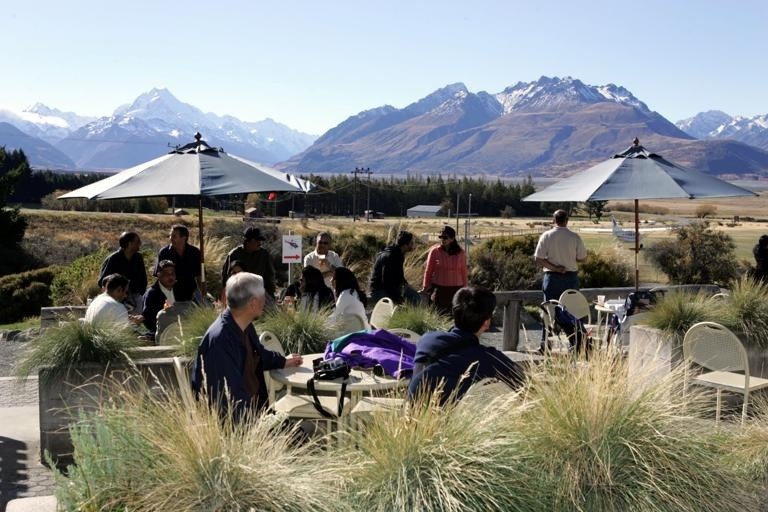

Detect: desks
[270, 353, 412, 451]
[595, 305, 615, 348]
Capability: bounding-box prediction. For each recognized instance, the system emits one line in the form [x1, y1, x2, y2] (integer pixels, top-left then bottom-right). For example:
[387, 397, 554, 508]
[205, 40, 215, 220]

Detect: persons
[533, 209, 588, 355]
[370, 231, 413, 306]
[409, 286, 530, 410]
[753, 235, 768, 288]
[84, 224, 202, 346]
[219, 226, 367, 314]
[422, 226, 468, 320]
[192, 273, 309, 450]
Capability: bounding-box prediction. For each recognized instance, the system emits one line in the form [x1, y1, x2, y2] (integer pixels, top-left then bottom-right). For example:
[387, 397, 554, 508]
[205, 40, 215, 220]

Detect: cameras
[312, 355, 351, 380]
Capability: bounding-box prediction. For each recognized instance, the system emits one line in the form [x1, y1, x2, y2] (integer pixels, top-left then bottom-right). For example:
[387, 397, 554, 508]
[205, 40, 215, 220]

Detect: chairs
[683, 322, 768, 434]
[159, 320, 191, 346]
[351, 397, 406, 465]
[370, 297, 393, 329]
[259, 331, 351, 455]
[387, 328, 419, 345]
[541, 300, 592, 361]
[174, 356, 199, 424]
[558, 289, 591, 325]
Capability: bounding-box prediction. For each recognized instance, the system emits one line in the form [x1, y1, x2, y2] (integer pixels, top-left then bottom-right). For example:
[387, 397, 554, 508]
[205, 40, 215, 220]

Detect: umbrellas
[520, 137, 761, 293]
[56, 132, 336, 281]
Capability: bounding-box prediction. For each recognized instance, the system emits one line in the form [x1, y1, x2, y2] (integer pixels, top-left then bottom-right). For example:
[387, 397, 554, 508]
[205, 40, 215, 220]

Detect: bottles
[163, 300, 170, 310]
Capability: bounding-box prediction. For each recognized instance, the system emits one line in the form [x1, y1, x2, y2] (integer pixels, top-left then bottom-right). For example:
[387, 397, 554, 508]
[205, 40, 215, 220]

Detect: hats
[439, 226, 455, 238]
[244, 227, 267, 241]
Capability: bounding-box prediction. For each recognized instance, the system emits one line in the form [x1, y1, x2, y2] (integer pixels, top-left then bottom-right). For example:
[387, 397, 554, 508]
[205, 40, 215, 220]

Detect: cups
[597, 295, 606, 305]
[318, 255, 326, 267]
[292, 354, 300, 366]
